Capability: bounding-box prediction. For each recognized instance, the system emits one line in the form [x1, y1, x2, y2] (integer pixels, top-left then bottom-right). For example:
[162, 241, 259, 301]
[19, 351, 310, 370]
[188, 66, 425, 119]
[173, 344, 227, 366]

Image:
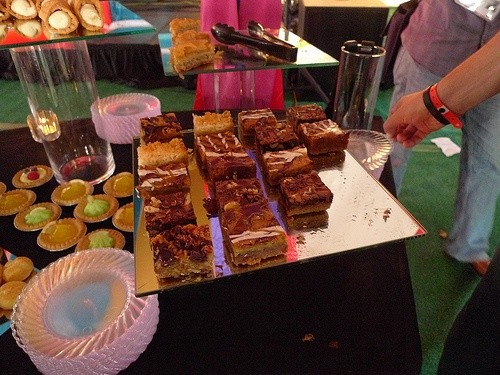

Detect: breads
[0, 248, 36, 309]
[0, 269, 37, 320]
[169, 17, 215, 72]
[137, 102, 348, 279]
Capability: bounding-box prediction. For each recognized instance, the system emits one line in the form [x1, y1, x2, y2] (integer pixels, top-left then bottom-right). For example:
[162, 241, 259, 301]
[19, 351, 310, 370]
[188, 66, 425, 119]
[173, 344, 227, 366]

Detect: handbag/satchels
[379, 0, 419, 90]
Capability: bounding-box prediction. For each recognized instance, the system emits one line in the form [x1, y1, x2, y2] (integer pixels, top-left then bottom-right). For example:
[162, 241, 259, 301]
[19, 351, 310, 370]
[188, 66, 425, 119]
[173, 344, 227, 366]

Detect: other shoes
[470, 258, 489, 273]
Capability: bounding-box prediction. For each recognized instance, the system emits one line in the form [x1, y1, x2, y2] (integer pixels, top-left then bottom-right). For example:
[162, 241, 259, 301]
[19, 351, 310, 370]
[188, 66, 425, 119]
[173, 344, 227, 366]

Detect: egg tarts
[0, 165, 134, 254]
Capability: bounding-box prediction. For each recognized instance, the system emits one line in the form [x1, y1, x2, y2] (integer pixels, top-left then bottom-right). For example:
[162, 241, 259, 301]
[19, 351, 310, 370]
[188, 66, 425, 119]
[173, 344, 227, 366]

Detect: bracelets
[422, 82, 463, 129]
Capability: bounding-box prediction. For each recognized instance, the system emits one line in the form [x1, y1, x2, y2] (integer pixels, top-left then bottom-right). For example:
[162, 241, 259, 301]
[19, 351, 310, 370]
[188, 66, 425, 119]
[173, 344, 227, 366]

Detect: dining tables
[0, 105, 424, 375]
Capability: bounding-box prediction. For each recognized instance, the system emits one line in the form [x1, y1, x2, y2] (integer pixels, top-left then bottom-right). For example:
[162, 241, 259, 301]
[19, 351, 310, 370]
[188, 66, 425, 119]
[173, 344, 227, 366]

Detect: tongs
[211, 19, 298, 62]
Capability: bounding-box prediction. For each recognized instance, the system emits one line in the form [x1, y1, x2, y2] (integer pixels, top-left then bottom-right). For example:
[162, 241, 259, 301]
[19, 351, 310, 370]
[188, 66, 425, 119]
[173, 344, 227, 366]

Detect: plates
[342, 129, 395, 171]
[10, 248, 160, 375]
[90, 93, 162, 144]
[0, 249, 40, 336]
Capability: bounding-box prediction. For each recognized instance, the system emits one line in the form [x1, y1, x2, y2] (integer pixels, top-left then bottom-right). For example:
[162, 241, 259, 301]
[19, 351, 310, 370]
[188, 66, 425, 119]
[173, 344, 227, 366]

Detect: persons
[189, 0, 284, 110]
[386, 0, 500, 276]
[382, 29, 500, 148]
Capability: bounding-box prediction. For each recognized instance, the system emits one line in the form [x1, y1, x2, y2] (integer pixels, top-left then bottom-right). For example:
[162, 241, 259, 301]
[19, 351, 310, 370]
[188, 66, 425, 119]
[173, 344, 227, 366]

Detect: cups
[9, 39, 116, 185]
[215, 71, 256, 127]
[333, 44, 387, 129]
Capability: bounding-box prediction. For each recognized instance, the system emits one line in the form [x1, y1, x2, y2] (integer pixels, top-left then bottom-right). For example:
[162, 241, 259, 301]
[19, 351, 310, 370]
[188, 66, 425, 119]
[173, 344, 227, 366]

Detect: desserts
[0, 18, 104, 43]
[0, 0, 105, 34]
